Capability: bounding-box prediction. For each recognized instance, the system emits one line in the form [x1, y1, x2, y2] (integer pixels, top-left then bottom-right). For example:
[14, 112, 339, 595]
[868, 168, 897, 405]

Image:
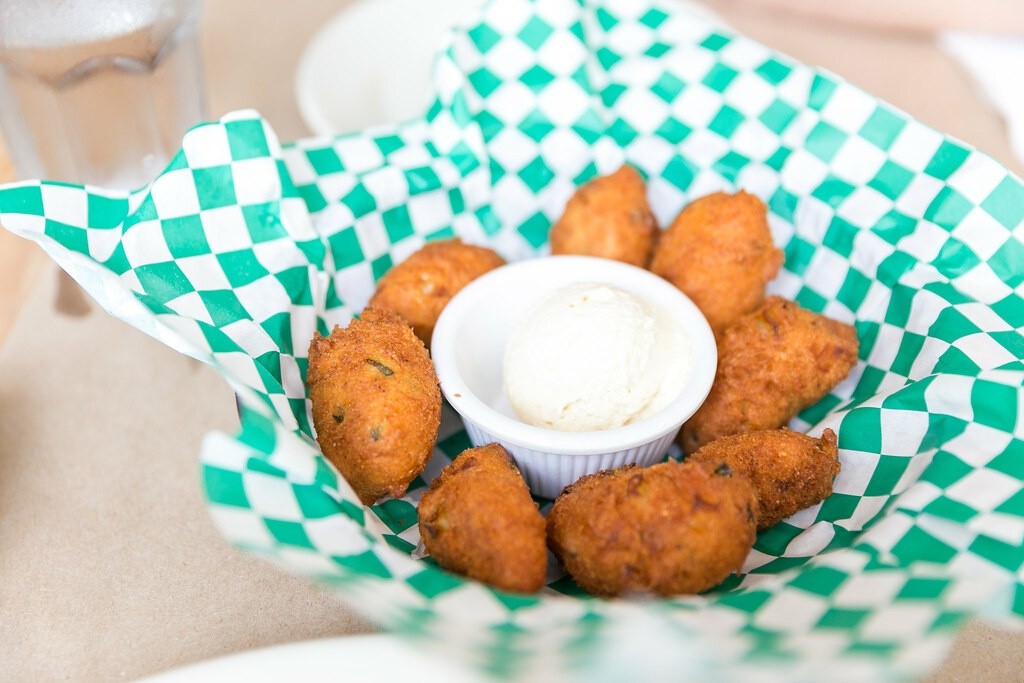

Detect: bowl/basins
[428, 254, 718, 498]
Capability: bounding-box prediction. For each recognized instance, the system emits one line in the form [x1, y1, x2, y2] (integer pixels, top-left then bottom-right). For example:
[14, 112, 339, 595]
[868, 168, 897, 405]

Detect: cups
[1, 0, 208, 189]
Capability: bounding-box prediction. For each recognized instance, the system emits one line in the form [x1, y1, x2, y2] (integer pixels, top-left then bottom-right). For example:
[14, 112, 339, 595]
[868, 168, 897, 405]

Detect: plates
[292, 3, 731, 146]
